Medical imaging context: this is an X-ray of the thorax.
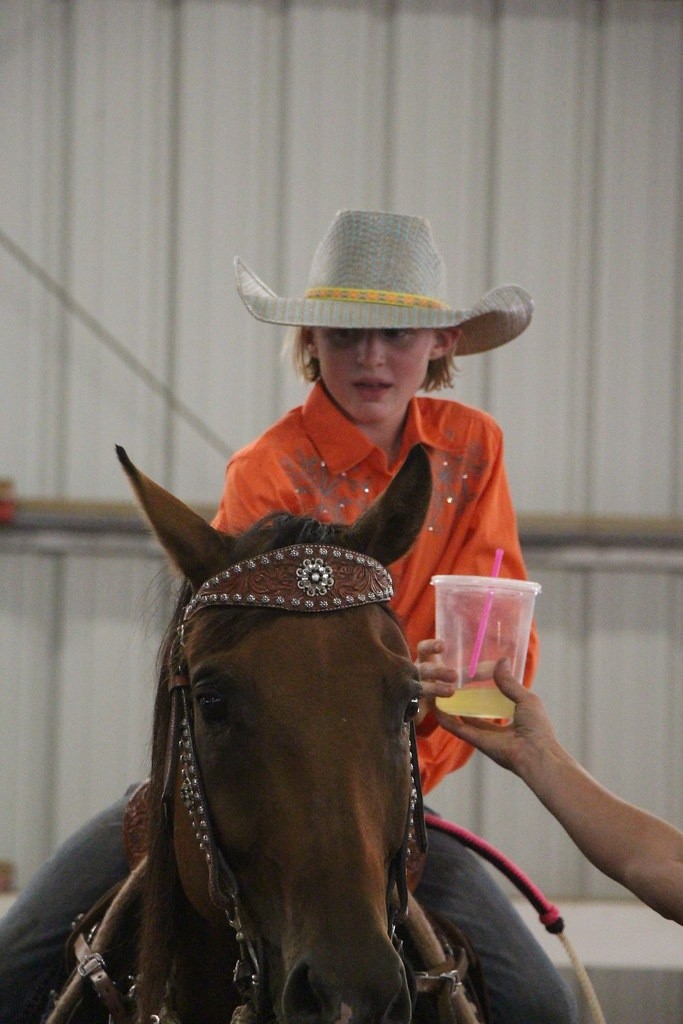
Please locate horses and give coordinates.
[37,445,485,1023]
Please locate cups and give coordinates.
[429,574,543,719]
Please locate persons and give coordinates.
[0,207,582,1024]
[426,658,683,929]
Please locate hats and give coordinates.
[234,212,534,357]
